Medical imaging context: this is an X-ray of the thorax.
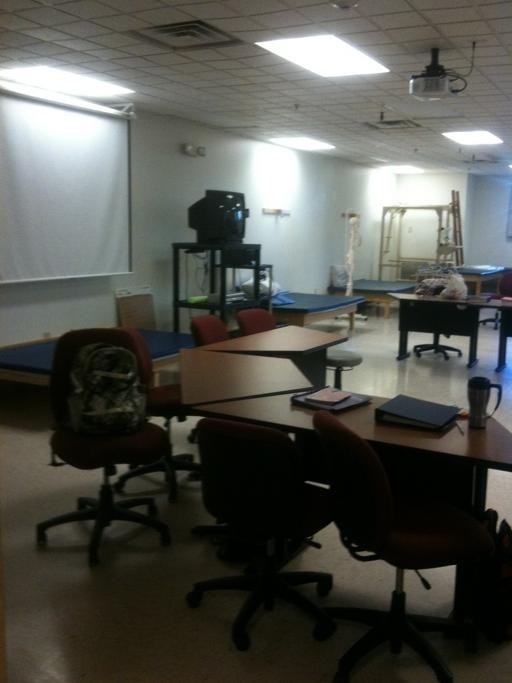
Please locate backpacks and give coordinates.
[68,343,145,435]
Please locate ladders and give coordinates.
[452,190,464,268]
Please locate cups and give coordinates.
[468,376,503,429]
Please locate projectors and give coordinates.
[407,76,454,102]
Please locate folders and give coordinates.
[375,395,463,431]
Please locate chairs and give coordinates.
[238,308,277,334]
[116,324,202,495]
[182,416,333,651]
[37,327,170,566]
[311,408,495,682]
[192,314,230,345]
[478,273,512,330]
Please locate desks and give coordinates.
[178,348,314,481]
[414,279,463,360]
[205,325,348,389]
[387,292,512,372]
[458,264,506,295]
[188,390,512,647]
[274,292,367,334]
[329,280,416,318]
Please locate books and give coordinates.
[307,384,352,405]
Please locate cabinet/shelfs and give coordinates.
[171,242,262,333]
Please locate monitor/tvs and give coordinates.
[187,189,250,243]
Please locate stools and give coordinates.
[324,350,364,390]
